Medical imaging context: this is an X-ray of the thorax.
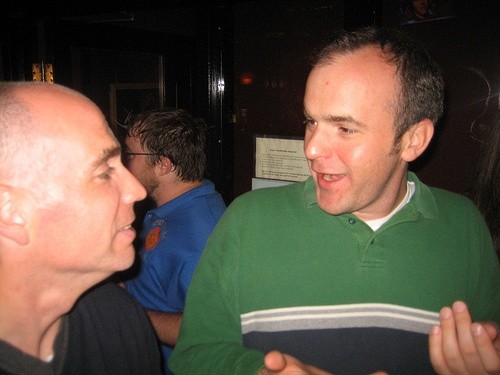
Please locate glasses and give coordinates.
[122,150,174,166]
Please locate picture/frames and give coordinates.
[107,81,164,140]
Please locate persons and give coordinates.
[0,80,167,375]
[165,27,500,375]
[118,108,227,360]
